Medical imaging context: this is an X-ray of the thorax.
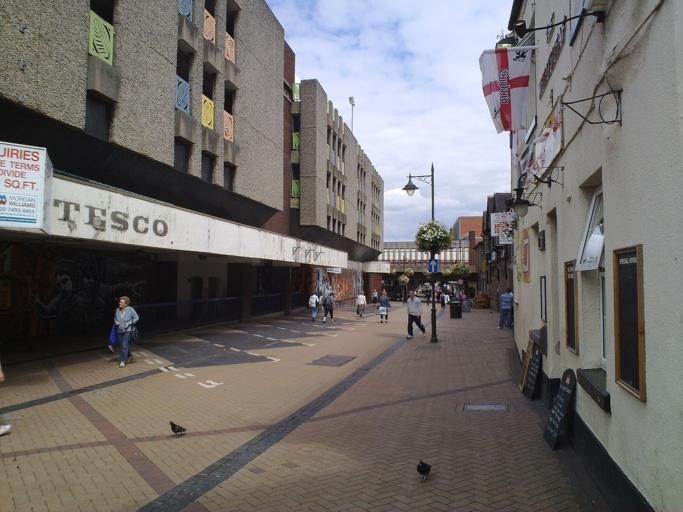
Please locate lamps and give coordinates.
[510,186,542,218]
[513,9,605,39]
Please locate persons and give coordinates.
[107,294,140,368]
[372,288,378,308]
[0,363,12,437]
[406,289,426,339]
[355,291,366,318]
[436,289,468,307]
[308,292,319,322]
[319,293,337,322]
[497,287,513,331]
[376,293,390,323]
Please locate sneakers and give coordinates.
[108,344,132,367]
[0,425,11,435]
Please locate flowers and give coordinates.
[415,219,451,255]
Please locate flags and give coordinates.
[477,46,535,135]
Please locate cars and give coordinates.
[419,283,439,296]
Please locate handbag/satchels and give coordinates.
[130,327,139,340]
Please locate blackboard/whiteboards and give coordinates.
[523,345,542,400]
[544,369,576,450]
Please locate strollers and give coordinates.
[460,300,469,312]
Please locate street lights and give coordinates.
[348,95,356,135]
[401,161,438,344]
[456,279,464,314]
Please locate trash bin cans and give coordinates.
[449,301,462,318]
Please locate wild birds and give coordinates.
[417,461,432,482]
[168,421,186,437]
